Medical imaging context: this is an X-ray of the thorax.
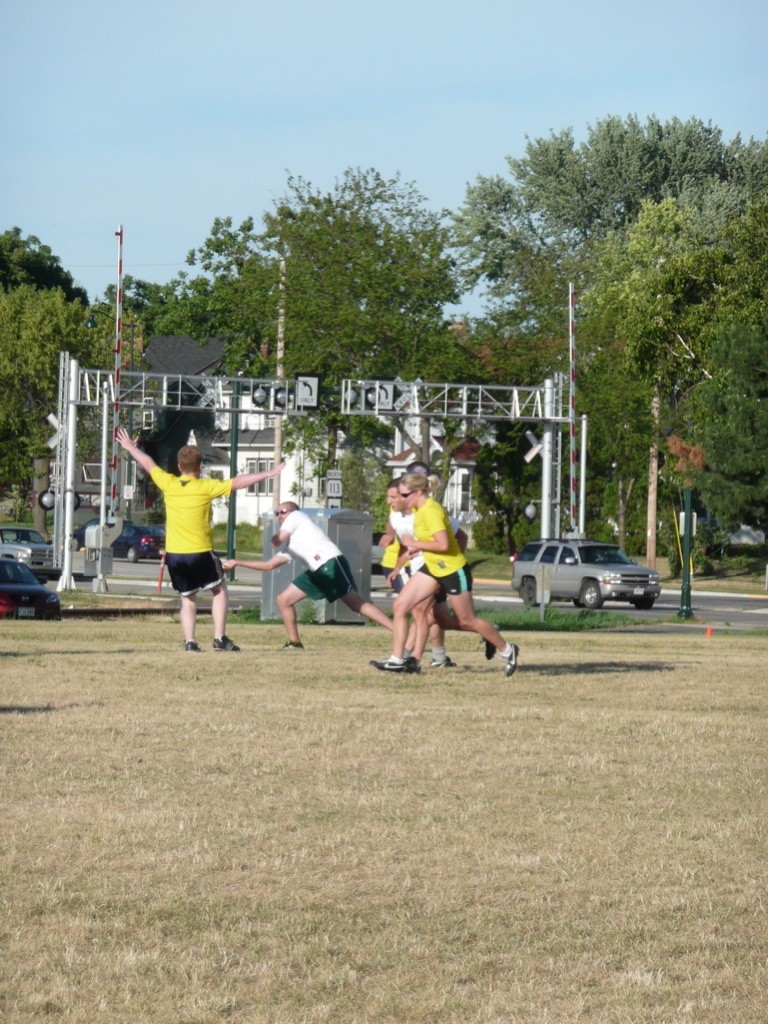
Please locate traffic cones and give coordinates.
[705,627,713,639]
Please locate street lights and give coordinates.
[86,308,139,520]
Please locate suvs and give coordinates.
[511,538,662,609]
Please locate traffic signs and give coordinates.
[296,375,318,407]
[374,382,392,410]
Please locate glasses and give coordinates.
[276,509,294,516]
[400,489,420,497]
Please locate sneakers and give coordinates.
[186,642,200,651]
[282,641,303,650]
[369,660,406,672]
[405,657,420,672]
[504,644,520,676]
[214,636,239,650]
[429,657,456,667]
[480,625,499,659]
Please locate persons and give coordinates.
[223,502,393,648]
[370,461,519,677]
[114,427,285,652]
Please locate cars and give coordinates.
[71,518,166,562]
[0,528,61,584]
[0,558,61,620]
[706,524,766,559]
